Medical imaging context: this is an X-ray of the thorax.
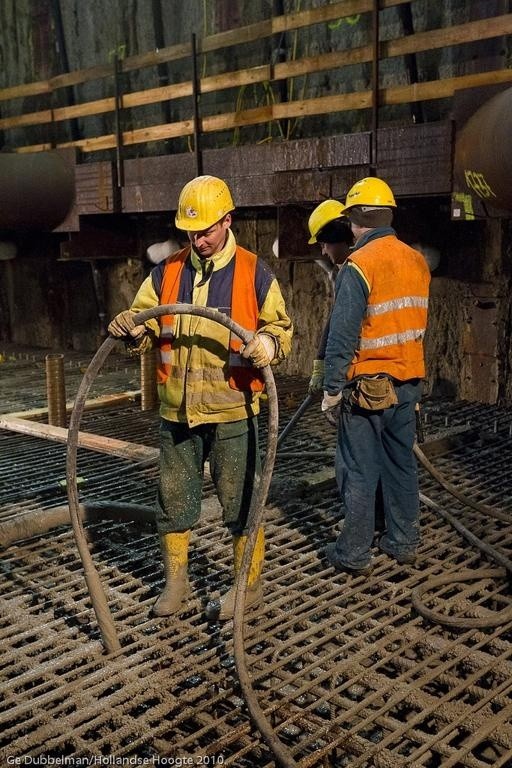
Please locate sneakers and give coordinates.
[372,530,417,564]
[325,542,373,577]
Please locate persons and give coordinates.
[104,173,297,621]
[317,174,430,574]
[302,196,395,505]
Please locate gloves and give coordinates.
[320,391,342,426]
[308,360,324,394]
[108,310,145,340]
[240,334,276,369]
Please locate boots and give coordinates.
[152,529,191,617]
[205,526,264,620]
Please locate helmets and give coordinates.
[308,199,345,245]
[340,176,397,214]
[174,175,235,232]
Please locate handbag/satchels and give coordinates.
[351,377,399,411]
